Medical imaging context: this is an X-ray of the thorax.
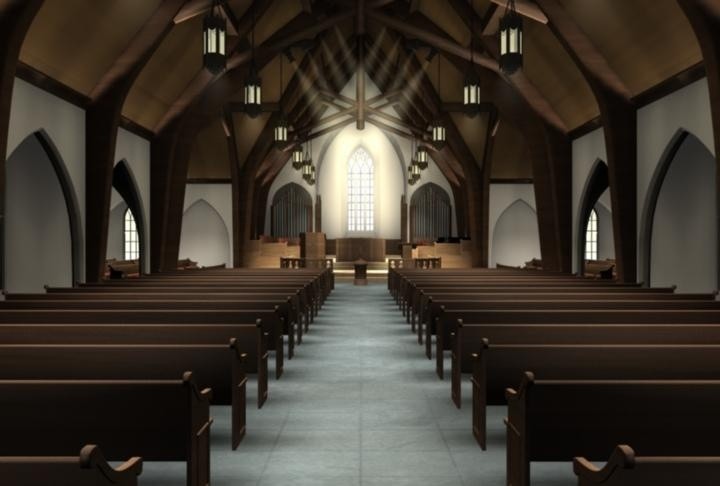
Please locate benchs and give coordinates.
[0,257,335,485]
[390,258,719,486]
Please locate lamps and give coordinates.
[293,87,316,185]
[204,2,228,78]
[272,48,289,147]
[244,1,262,118]
[464,11,482,118]
[499,0,523,76]
[432,53,448,149]
[407,90,429,186]
